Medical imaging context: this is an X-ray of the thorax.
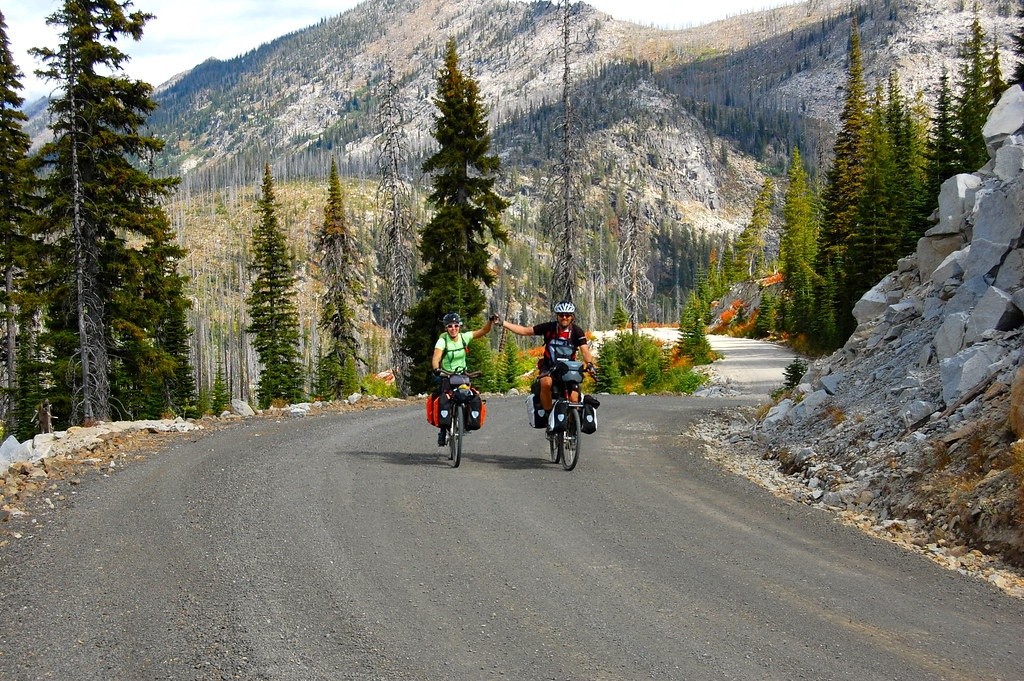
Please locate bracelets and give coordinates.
[587,362,593,365]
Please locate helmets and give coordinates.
[553,301,575,313]
[443,313,460,325]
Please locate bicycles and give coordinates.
[426,368,486,468]
[526,360,601,472]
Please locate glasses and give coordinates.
[557,312,573,318]
[445,324,459,328]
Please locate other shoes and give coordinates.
[437,430,447,446]
[545,425,556,440]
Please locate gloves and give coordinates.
[431,368,441,378]
[489,313,498,321]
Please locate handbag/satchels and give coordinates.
[525,393,548,428]
[581,393,600,434]
[426,390,452,429]
[462,387,486,431]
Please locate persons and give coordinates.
[494,301,596,450]
[431,312,499,446]
